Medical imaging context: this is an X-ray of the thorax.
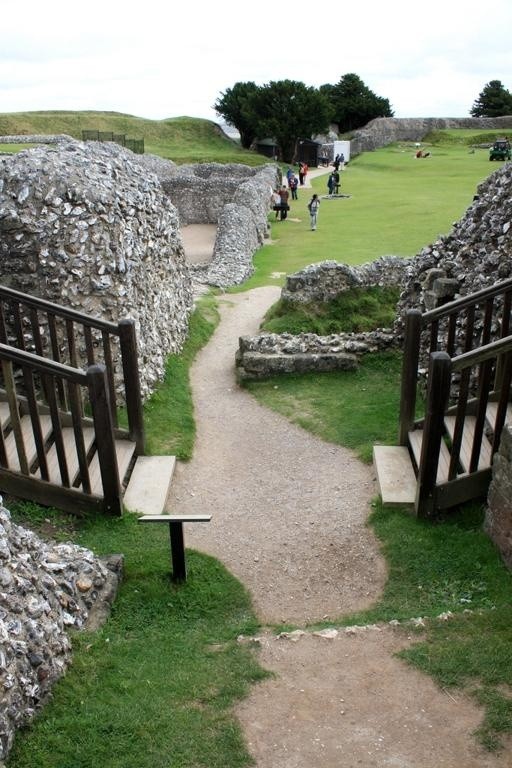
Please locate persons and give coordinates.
[271,161,308,221]
[327,153,345,194]
[307,194,321,231]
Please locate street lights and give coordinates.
[290,95,296,135]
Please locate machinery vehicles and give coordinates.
[489,139,512,160]
